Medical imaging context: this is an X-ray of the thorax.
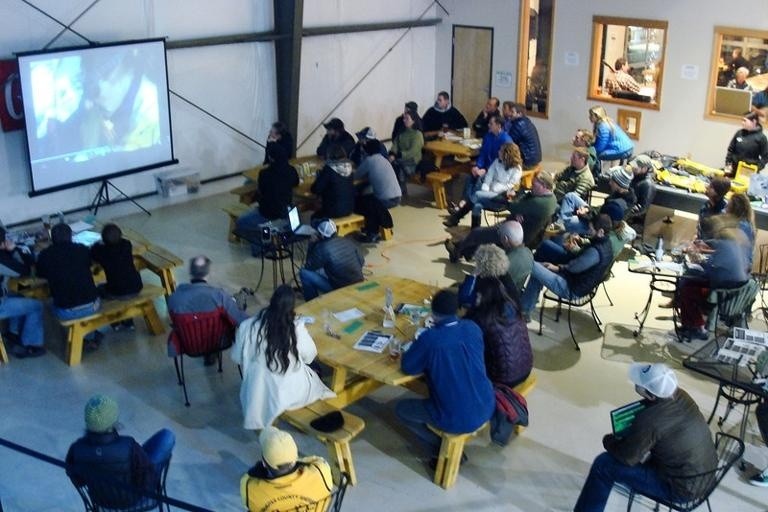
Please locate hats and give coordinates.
[84,393,120,434]
[311,218,338,239]
[256,425,298,474]
[629,154,653,170]
[612,164,634,190]
[628,361,679,398]
[321,118,345,131]
[600,200,625,222]
[355,126,376,141]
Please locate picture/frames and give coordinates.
[588,17,670,110]
[705,25,768,131]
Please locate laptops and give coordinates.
[288,206,319,236]
[611,399,659,463]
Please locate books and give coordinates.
[715,326,768,379]
[68,221,94,234]
[352,331,394,354]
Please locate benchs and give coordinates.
[231,182,257,205]
[426,374,538,489]
[122,226,183,297]
[0,334,10,363]
[282,400,365,486]
[415,168,452,209]
[330,213,391,241]
[521,164,540,189]
[59,284,166,366]
[224,201,253,243]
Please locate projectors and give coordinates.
[258,223,273,245]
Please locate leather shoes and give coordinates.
[2,329,22,347]
[442,216,458,227]
[83,330,101,352]
[447,206,458,216]
[15,344,46,359]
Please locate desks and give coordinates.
[425,130,483,158]
[627,243,703,342]
[293,275,468,385]
[10,218,145,294]
[683,334,768,471]
[643,159,768,230]
[244,156,367,198]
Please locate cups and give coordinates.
[429,279,438,301]
[389,339,401,361]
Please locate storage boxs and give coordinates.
[154,166,200,198]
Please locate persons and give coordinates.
[724,111,768,176]
[695,176,731,241]
[606,59,641,96]
[229,284,339,430]
[240,426,334,512]
[502,101,513,125]
[573,363,718,512]
[235,145,299,259]
[498,221,535,297]
[629,155,656,219]
[522,213,614,324]
[262,121,294,165]
[589,105,634,161]
[388,111,425,206]
[64,49,161,163]
[443,143,523,230]
[391,101,440,184]
[446,116,514,217]
[748,401,768,489]
[674,216,749,341]
[463,279,534,386]
[352,139,402,242]
[65,394,176,509]
[421,91,469,141]
[533,201,623,264]
[658,193,756,315]
[396,290,496,471]
[563,199,637,242]
[36,224,102,352]
[472,98,500,140]
[470,243,522,320]
[444,169,557,263]
[316,118,356,159]
[0,228,46,359]
[508,103,542,171]
[350,126,389,196]
[727,66,752,93]
[299,218,364,302]
[166,256,244,368]
[572,129,602,185]
[90,224,143,333]
[553,147,595,205]
[545,165,635,233]
[752,86,768,117]
[724,48,753,80]
[310,144,354,223]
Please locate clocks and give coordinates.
[518,0,556,119]
[616,109,641,140]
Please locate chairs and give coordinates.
[713,279,756,346]
[169,313,243,407]
[590,249,623,325]
[74,453,172,512]
[286,473,350,512]
[483,207,509,226]
[538,256,615,350]
[625,433,745,511]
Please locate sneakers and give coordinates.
[202,359,218,367]
[238,286,251,312]
[113,323,137,332]
[428,452,468,471]
[751,470,768,489]
[678,326,710,341]
[353,231,380,242]
[446,236,471,263]
